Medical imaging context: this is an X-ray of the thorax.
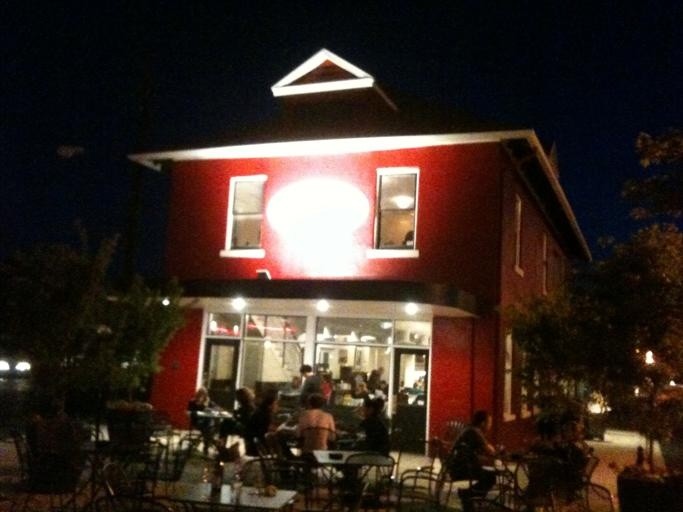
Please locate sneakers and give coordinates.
[458,488,471,509]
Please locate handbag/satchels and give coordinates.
[218,441,240,462]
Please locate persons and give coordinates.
[446,409,505,512]
[184,387,225,448]
[215,386,255,454]
[244,361,392,504]
[521,414,590,511]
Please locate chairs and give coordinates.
[6,403,601,511]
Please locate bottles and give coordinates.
[211,457,224,491]
